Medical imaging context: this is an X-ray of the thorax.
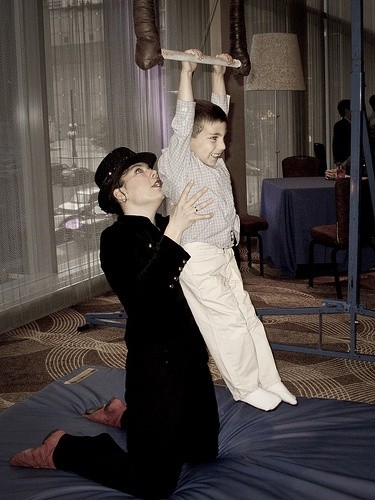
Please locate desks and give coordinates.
[258,176,375,278]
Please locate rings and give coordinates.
[195,206,198,211]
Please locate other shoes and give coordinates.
[84,397,126,428]
[10,428,66,469]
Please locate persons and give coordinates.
[369,95,375,137]
[157,49,297,410]
[332,99,351,175]
[8,146,219,500]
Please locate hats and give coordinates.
[93,146,157,218]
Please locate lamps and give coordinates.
[244,32,306,178]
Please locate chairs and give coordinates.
[230,178,268,276]
[280,156,324,179]
[308,178,375,300]
